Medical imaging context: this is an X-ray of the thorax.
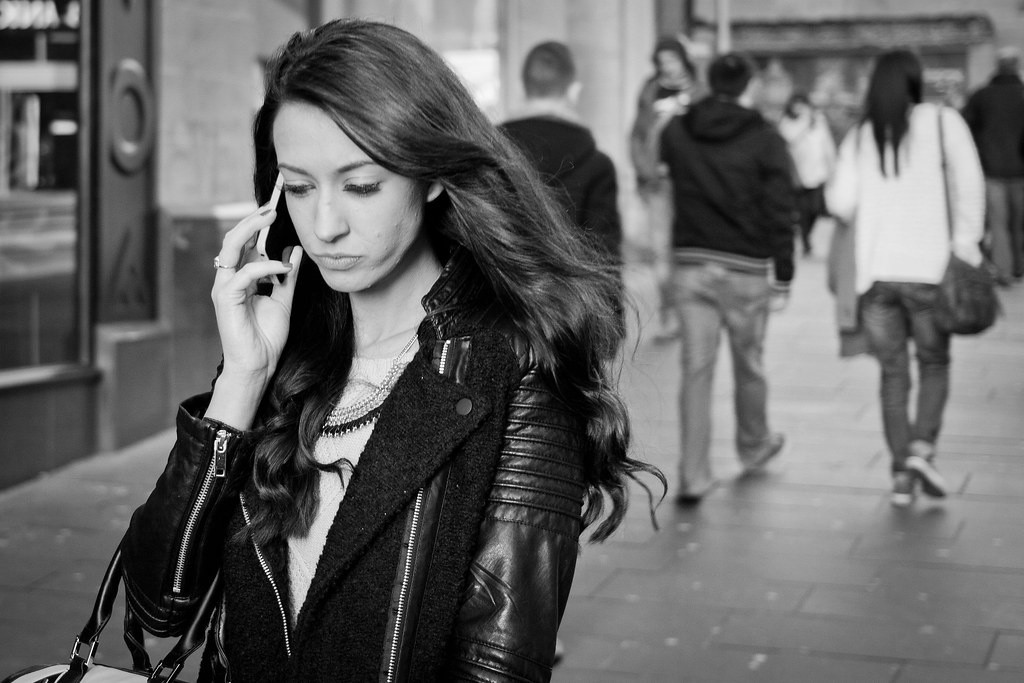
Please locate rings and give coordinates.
[214,257,239,270]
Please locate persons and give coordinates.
[823,50,987,505]
[124,19,636,683]
[658,54,794,505]
[488,42,626,362]
[960,46,1024,282]
[631,34,713,345]
[779,94,836,257]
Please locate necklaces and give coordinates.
[319,335,419,437]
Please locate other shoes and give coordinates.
[741,431,785,474]
[890,476,918,506]
[677,478,718,506]
[905,444,950,496]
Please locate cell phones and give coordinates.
[257,171,300,287]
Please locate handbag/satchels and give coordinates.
[3,512,218,683]
[936,251,1003,331]
